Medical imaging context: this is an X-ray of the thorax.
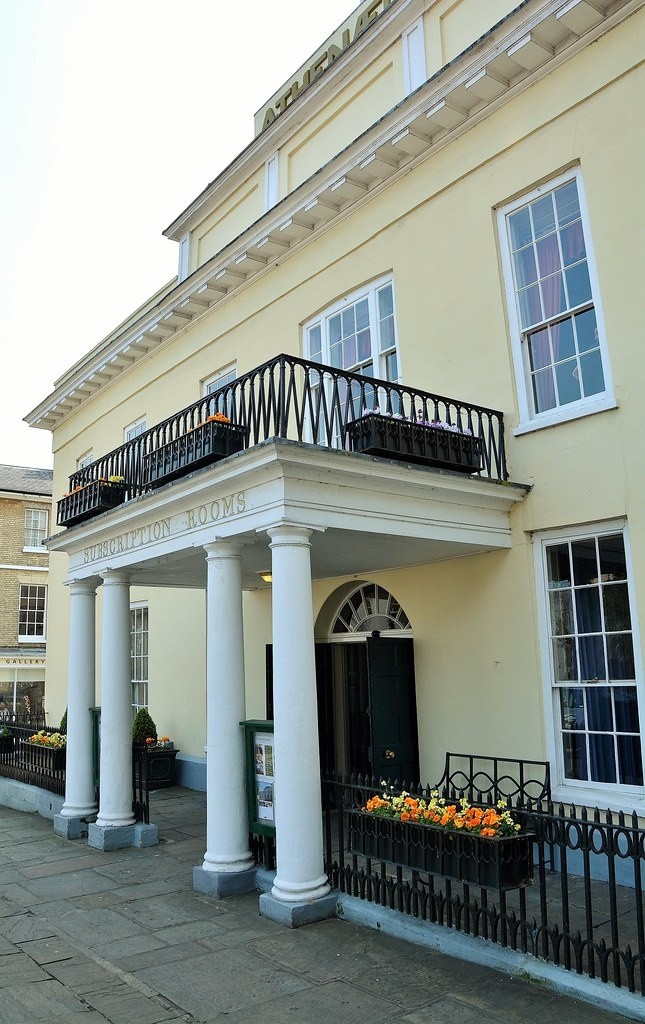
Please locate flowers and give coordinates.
[143,736,173,751]
[63,475,125,498]
[360,404,472,436]
[168,412,231,451]
[26,730,66,749]
[362,781,522,837]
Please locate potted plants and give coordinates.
[0,723,14,754]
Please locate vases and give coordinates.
[346,413,485,473]
[141,419,249,489]
[56,479,131,527]
[347,808,535,892]
[19,741,66,771]
[134,750,180,790]
